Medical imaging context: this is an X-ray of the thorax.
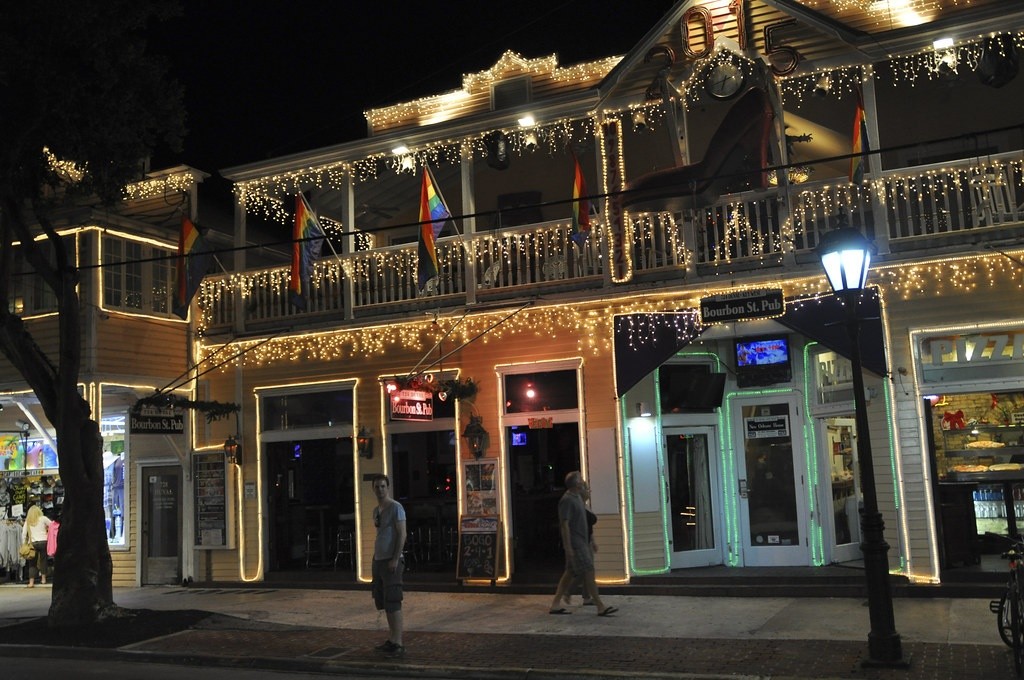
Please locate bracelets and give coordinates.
[799,135,802,143]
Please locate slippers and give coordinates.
[584,600,596,605]
[376,641,404,652]
[598,607,618,616]
[549,609,572,615]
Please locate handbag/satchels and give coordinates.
[20,543,36,560]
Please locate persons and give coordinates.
[564,480,599,605]
[42,440,58,467]
[26,442,42,468]
[47,510,62,555]
[548,472,619,615]
[22,504,52,587]
[112,452,124,542]
[768,124,814,163]
[14,445,25,470]
[103,451,119,543]
[371,473,406,658]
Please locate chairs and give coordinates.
[403,527,458,563]
[304,533,354,571]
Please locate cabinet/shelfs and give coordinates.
[943,427,1024,482]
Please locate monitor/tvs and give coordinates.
[733,335,793,388]
[512,433,526,446]
[667,372,726,409]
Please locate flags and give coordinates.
[758,75,775,191]
[288,187,325,311]
[417,166,449,291]
[848,87,869,194]
[176,215,199,308]
[569,160,591,248]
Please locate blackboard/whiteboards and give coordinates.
[456,514,500,580]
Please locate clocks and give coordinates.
[705,60,746,100]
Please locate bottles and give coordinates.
[970,486,1024,518]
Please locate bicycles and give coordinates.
[985,531,1024,680]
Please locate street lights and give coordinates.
[815,201,914,669]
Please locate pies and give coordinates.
[967,441,1005,447]
[953,463,1021,471]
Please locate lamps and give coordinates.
[357,426,373,460]
[439,387,489,461]
[16,419,29,431]
[224,435,242,465]
